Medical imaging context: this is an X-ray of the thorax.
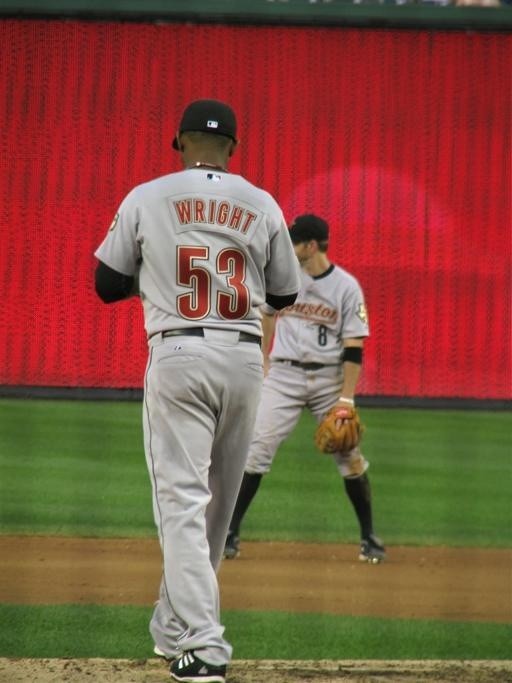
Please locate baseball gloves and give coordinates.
[315,404,362,454]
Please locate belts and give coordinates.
[162,328,261,344]
[277,360,325,370]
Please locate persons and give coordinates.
[93,99,302,682]
[223,212,387,565]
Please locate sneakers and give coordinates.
[221,531,241,559]
[153,645,184,662]
[359,534,386,564]
[169,649,226,683]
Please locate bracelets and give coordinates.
[339,396,354,406]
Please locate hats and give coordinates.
[173,100,237,152]
[289,215,328,240]
